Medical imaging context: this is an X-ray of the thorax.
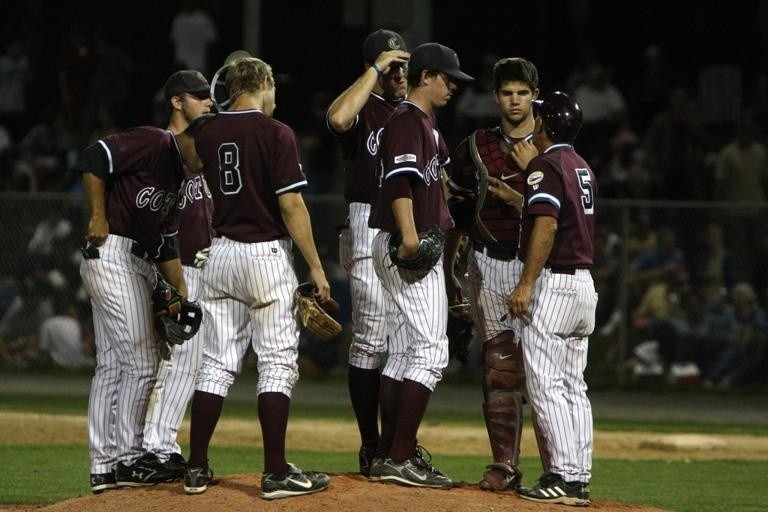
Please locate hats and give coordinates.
[410,42,476,83]
[613,132,636,145]
[163,70,211,103]
[362,29,408,66]
[532,90,585,143]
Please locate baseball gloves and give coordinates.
[154,286,201,345]
[389,229,444,270]
[294,284,343,339]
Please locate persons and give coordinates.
[436,236,488,388]
[325,27,456,477]
[375,44,466,490]
[183,57,333,497]
[445,55,570,492]
[507,97,597,505]
[293,254,352,378]
[585,66,768,388]
[0,32,121,369]
[141,72,222,477]
[77,120,193,493]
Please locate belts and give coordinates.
[471,241,517,261]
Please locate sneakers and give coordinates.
[89,470,116,493]
[183,464,214,494]
[116,453,179,487]
[162,453,187,481]
[357,443,457,490]
[476,462,590,507]
[259,463,330,500]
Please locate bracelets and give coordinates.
[369,63,386,82]
